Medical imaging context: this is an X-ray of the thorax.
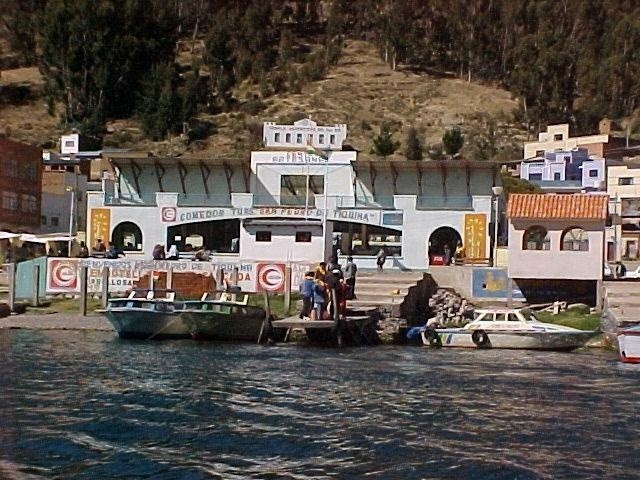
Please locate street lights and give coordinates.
[492,186,503,267]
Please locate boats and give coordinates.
[93,288,266,341]
[419,307,604,349]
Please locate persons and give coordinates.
[455,240,466,258]
[153,242,209,261]
[444,245,452,266]
[77,239,118,258]
[377,248,386,271]
[299,255,358,320]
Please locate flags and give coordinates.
[307,140,329,161]
